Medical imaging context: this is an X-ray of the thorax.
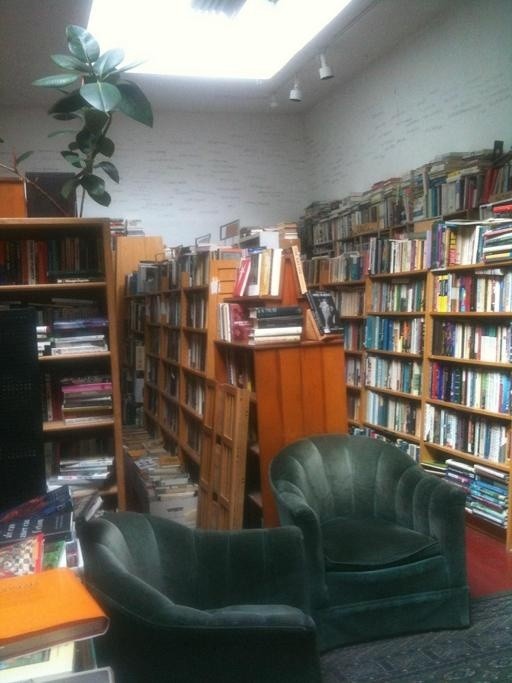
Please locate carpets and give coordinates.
[324,591,512,683]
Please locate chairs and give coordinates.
[268,434,471,648]
[77,510,321,683]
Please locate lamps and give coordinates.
[319,52,334,80]
[289,77,302,102]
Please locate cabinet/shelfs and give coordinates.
[0,162,512,557]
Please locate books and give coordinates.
[1,149,512,682]
